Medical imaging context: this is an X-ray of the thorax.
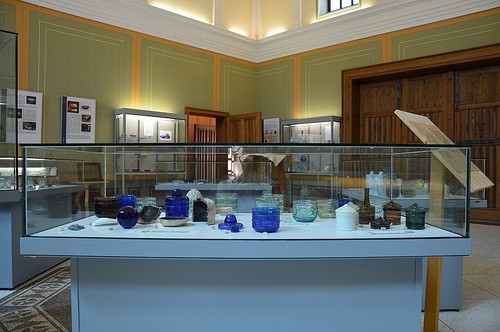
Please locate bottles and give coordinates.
[192,197,208,221]
[376,171,384,196]
[359,187,375,224]
[165,188,190,217]
[366,171,378,195]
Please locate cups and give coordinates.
[292,199,318,222]
[252,193,284,233]
[120,194,136,207]
[214,192,239,216]
[93,196,120,218]
